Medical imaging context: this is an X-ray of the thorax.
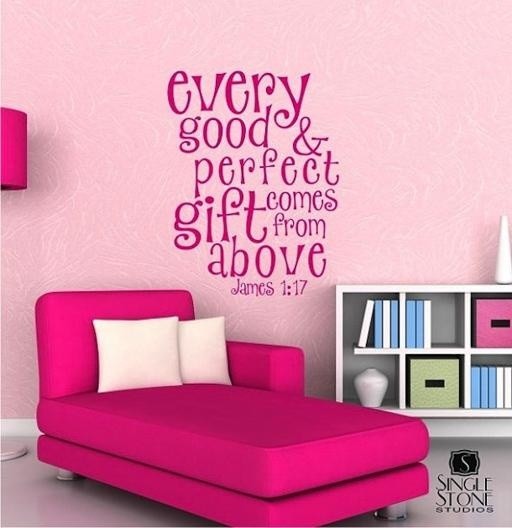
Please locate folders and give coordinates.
[407,298,430,348]
[357,299,397,348]
[471,366,511,408]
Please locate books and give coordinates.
[357,299,432,352]
[471,364,512,408]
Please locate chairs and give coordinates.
[34,288,431,527]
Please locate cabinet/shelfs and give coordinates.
[335,286,512,522]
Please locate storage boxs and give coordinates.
[473,299,512,348]
[409,358,460,409]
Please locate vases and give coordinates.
[354,368,389,408]
[495,216,511,284]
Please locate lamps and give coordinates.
[0,107,28,462]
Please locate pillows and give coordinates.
[92,315,184,394]
[178,316,233,386]
